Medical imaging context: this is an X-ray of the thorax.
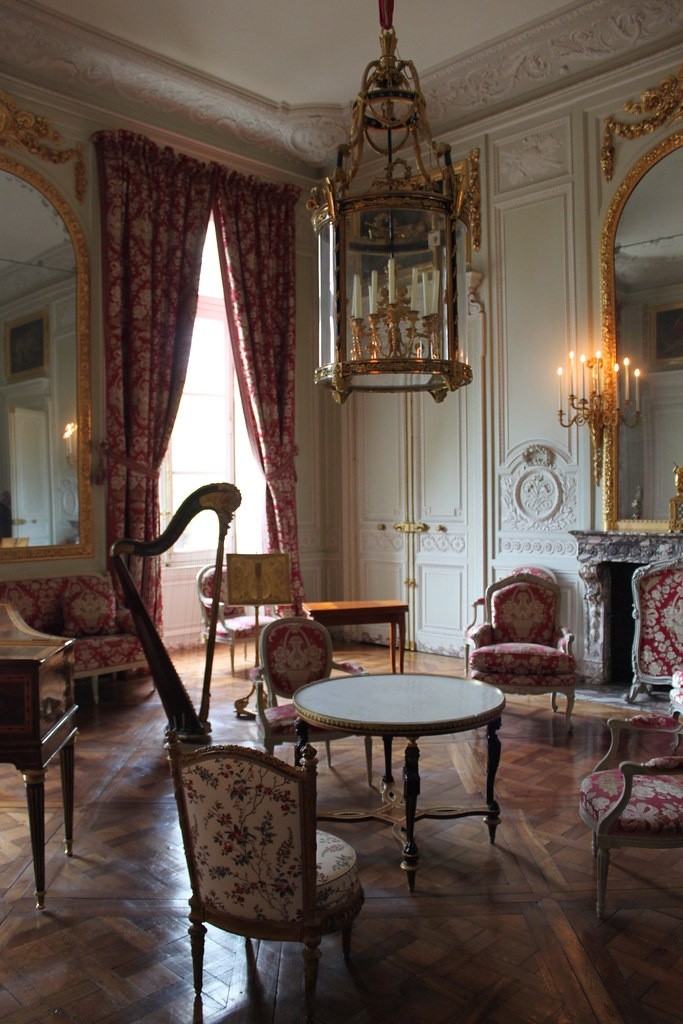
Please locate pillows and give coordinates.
[61,589,120,637]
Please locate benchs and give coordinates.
[0,572,157,705]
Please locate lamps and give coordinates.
[556,349,641,487]
[63,421,80,467]
[305,0,473,403]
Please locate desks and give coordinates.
[302,600,409,674]
[292,673,506,895]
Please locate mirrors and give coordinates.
[600,128,683,535]
[1,157,94,562]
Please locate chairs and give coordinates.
[254,616,373,787]
[196,564,283,678]
[464,564,577,733]
[164,730,366,1024]
[580,711,683,918]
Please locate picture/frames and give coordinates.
[356,159,469,287]
[4,305,49,380]
[652,302,683,365]
